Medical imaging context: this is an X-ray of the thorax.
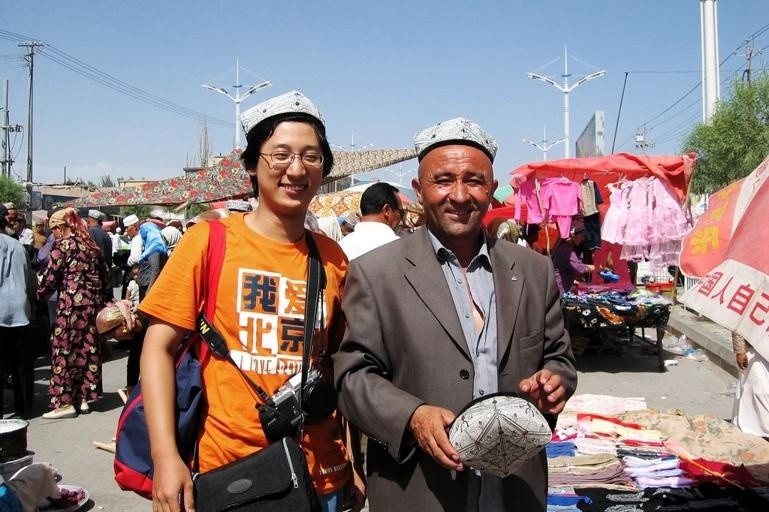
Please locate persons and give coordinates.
[186,200,253,233]
[0,200,185,422]
[330,119,578,511]
[136,89,368,512]
[549,226,604,297]
[303,177,532,265]
[730,329,769,442]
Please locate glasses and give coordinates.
[259,152,325,166]
[392,207,404,217]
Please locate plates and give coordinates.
[41,483,88,511]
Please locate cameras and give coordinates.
[258,368,338,445]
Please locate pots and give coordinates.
[0,417,31,458]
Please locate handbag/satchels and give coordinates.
[182,438,324,512]
[114,346,204,501]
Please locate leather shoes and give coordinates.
[77,403,90,415]
[43,405,77,419]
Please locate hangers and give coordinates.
[604,172,627,189]
[582,170,588,182]
[560,170,569,182]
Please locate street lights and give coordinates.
[522,125,568,160]
[529,44,608,160]
[200,61,272,157]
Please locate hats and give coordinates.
[3,200,251,232]
[448,393,553,478]
[240,90,327,143]
[413,118,497,164]
